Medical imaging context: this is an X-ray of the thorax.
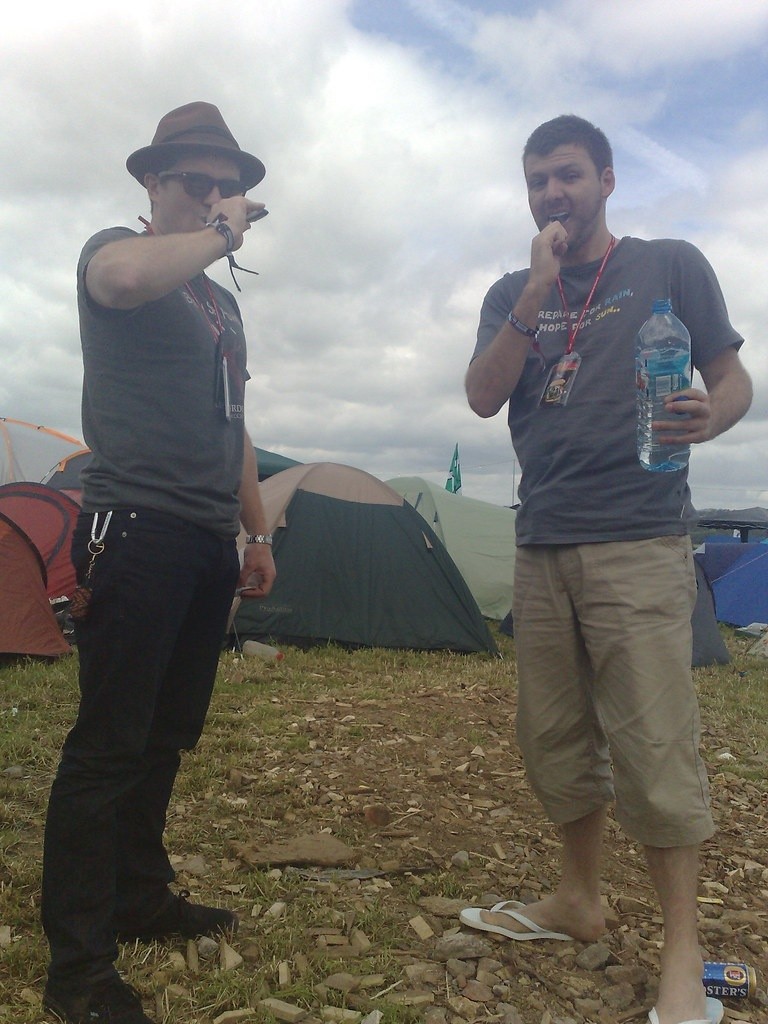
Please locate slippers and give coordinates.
[645,997,725,1024]
[458,900,576,941]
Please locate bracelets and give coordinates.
[208,213,260,294]
[246,534,273,544]
[507,311,539,339]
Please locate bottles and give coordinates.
[635,298,691,473]
[243,640,283,661]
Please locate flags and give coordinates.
[445,443,462,494]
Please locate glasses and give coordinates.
[157,170,251,199]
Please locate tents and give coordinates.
[0,416,768,671]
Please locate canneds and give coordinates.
[701,961,756,998]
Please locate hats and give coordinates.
[125,100,266,192]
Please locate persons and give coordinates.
[44,103,278,1022]
[465,116,752,1023]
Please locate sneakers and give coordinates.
[40,973,158,1024]
[110,888,240,946]
